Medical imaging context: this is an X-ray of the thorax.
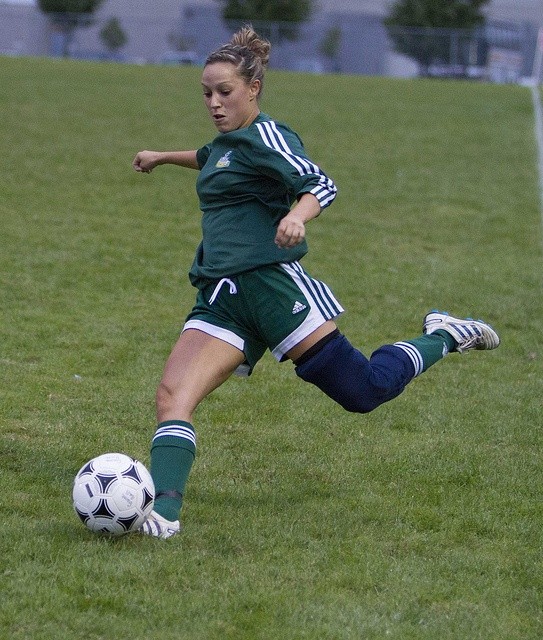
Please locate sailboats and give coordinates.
[72,453,155,536]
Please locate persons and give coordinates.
[130,22,501,540]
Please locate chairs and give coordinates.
[422,308,500,354]
[136,511,181,540]
